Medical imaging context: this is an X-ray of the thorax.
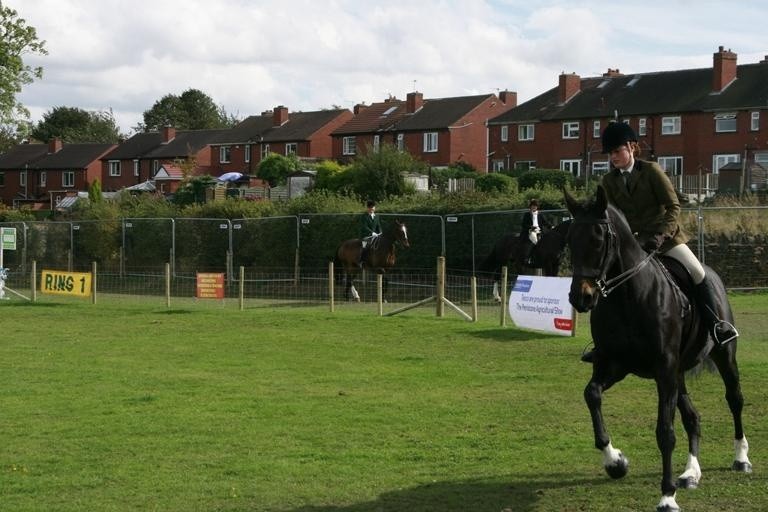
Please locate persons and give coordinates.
[359,201,383,264]
[519,200,554,264]
[579,123,739,362]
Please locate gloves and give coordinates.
[644,234,661,251]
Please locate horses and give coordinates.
[559,183,754,512]
[480,217,574,304]
[333,218,412,304]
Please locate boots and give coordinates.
[696,275,736,338]
[358,248,368,267]
[579,348,599,362]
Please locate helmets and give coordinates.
[529,200,539,207]
[367,200,377,207]
[601,123,638,153]
